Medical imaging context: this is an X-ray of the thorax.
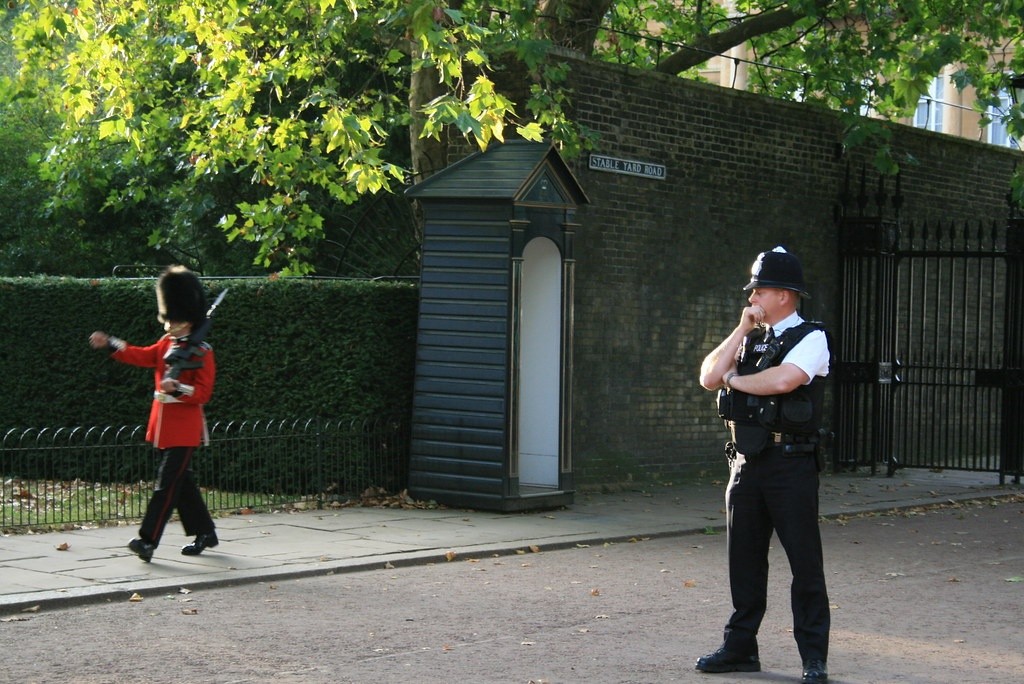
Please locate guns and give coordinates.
[807,435,827,472]
[160,286,231,398]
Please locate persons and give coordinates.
[89,321,218,562]
[695,252,837,684]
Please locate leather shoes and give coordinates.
[800,658,827,684]
[696,645,762,672]
[130,539,153,562]
[181,530,218,556]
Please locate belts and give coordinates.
[772,433,819,444]
[154,390,181,404]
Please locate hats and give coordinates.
[157,266,206,331]
[742,248,811,300]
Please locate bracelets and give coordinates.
[727,373,739,385]
